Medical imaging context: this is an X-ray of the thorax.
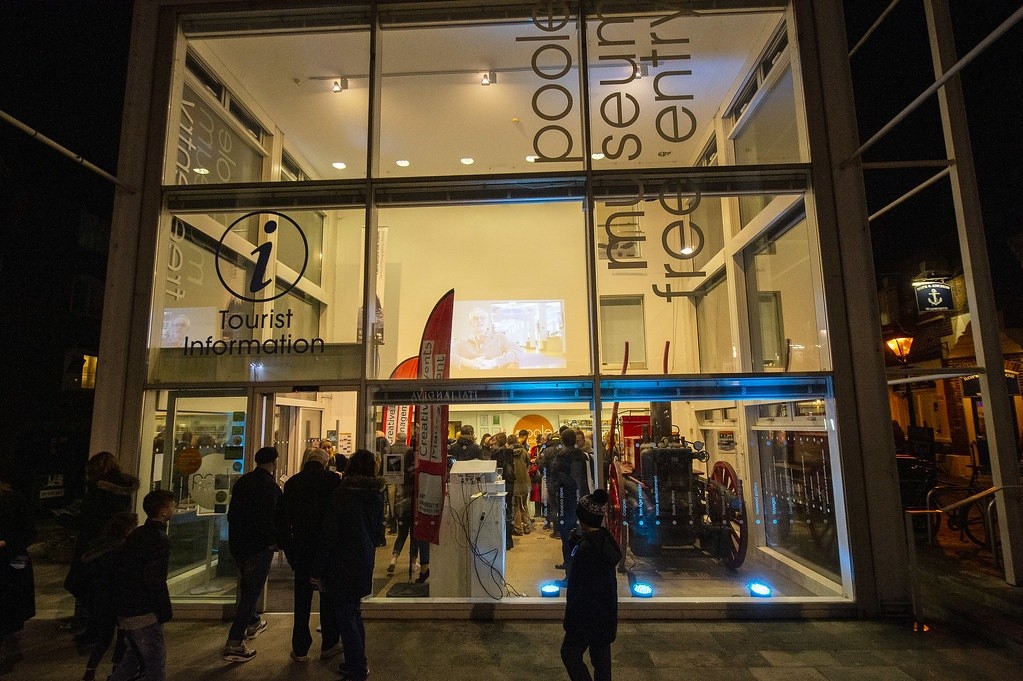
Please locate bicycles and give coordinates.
[911,460,1003,549]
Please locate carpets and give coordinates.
[268,578,391,611]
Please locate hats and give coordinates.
[575,489,609,528]
[306,448,329,461]
[255,446,279,464]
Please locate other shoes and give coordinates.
[337,672,367,681]
[554,577,568,588]
[415,567,430,583]
[337,662,369,675]
[320,643,344,659]
[290,649,308,662]
[72,628,106,651]
[543,523,562,539]
[554,562,566,569]
[511,518,537,536]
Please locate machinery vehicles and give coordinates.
[609,339,750,569]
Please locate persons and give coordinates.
[0,450,174,681]
[559,489,621,681]
[150,423,231,513]
[453,307,523,369]
[220,439,390,681]
[892,419,905,452]
[377,424,622,585]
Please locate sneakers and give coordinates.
[409,562,421,571]
[386,561,396,573]
[223,640,257,663]
[246,620,268,639]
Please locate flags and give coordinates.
[382,353,421,447]
[414,287,454,545]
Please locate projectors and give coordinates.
[450,460,498,484]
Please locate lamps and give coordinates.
[634,64,649,79]
[540,585,560,597]
[333,77,349,93]
[482,71,497,86]
[632,583,653,598]
[749,583,772,597]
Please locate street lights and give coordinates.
[887,323,917,425]
